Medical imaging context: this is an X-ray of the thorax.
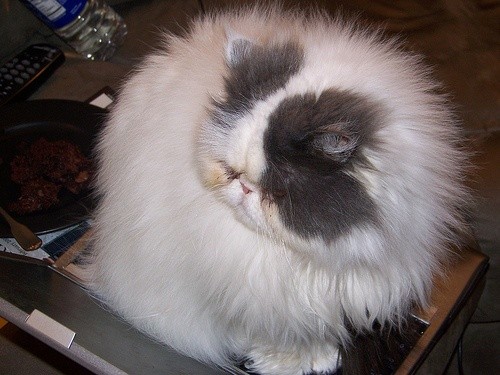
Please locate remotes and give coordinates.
[1,43,65,111]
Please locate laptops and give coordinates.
[0,222,488,375]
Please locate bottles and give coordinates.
[20,0,129,64]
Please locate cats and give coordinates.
[60,0,483,375]
